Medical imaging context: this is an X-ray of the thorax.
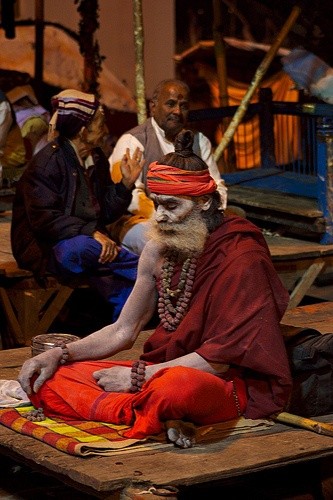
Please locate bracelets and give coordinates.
[25,407,46,422]
[130,360,148,392]
[52,341,70,365]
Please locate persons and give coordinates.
[17,130,293,448]
[11,89,147,324]
[109,78,228,257]
[0,88,26,190]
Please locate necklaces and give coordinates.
[158,251,197,332]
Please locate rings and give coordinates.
[136,158,141,163]
